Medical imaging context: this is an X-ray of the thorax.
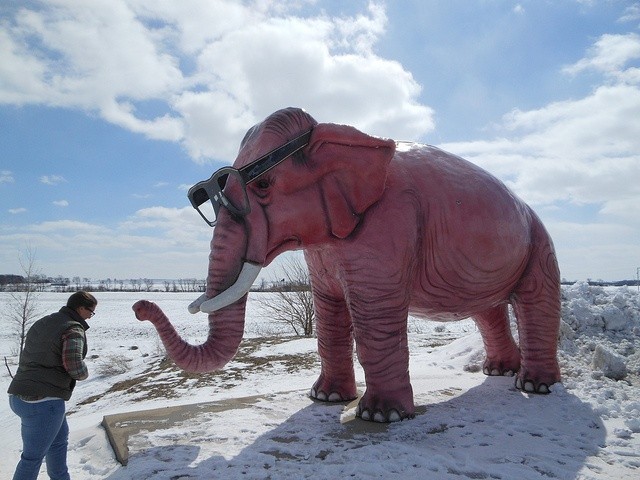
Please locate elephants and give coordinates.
[132,107,561,423]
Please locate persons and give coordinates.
[7,289,98,480]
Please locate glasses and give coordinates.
[86,308,96,317]
